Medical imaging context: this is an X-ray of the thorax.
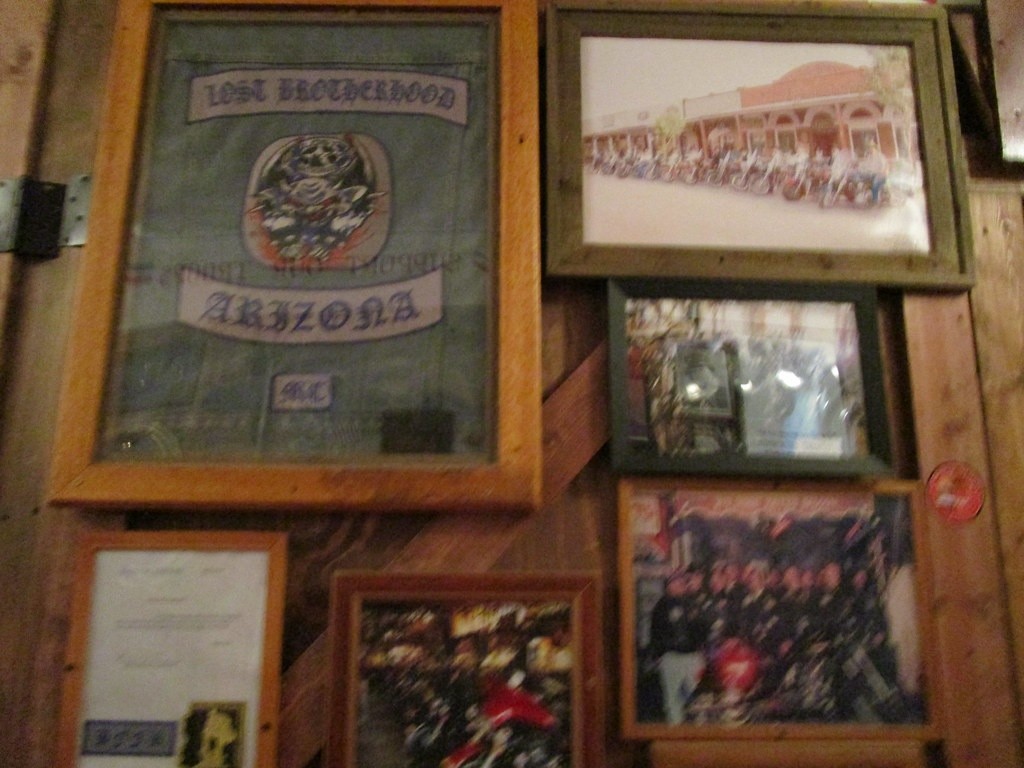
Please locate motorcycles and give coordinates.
[371,656,571,767]
[586,145,893,211]
[707,634,842,724]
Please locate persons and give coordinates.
[649,509,927,726]
[365,604,573,768]
[589,139,890,207]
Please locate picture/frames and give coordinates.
[43,0,547,512]
[327,567,604,768]
[58,532,288,767]
[619,481,946,742]
[540,8,978,297]
[600,277,892,479]
